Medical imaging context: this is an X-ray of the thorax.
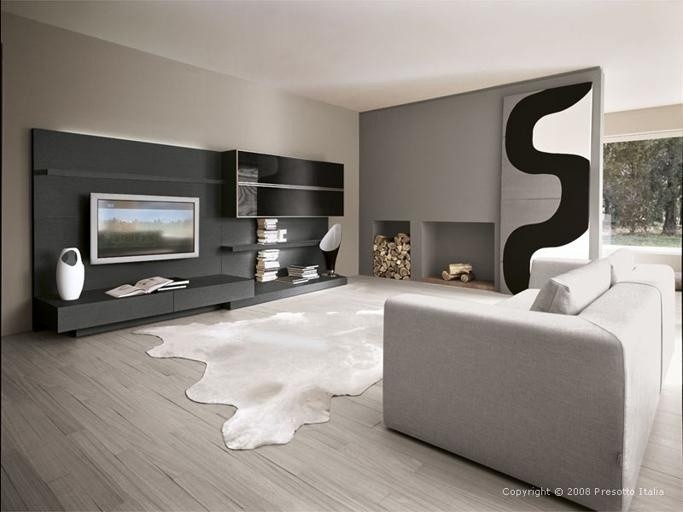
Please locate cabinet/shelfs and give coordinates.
[34,273,254,339]
[222,148,345,220]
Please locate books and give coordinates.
[105,275,190,299]
[287,264,319,285]
[254,219,286,281]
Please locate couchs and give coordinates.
[383,259,675,512]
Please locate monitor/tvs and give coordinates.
[90,193,200,265]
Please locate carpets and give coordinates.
[131,310,384,452]
